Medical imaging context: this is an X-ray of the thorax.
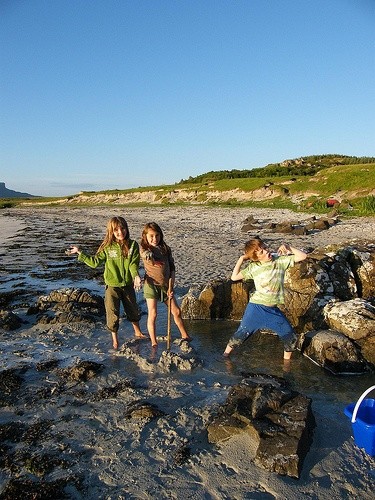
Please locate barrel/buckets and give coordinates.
[343,385,375,457]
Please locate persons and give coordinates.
[225,239,307,359]
[140,222,191,346]
[65,217,146,347]
